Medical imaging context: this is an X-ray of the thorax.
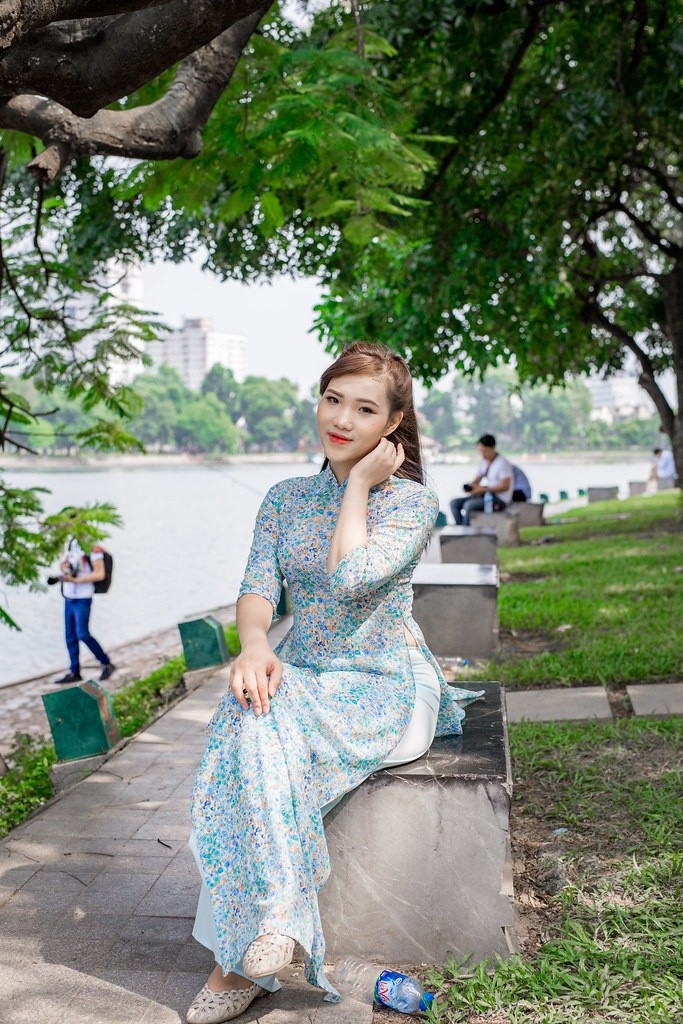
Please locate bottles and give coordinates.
[484,491,493,513]
[333,956,435,1013]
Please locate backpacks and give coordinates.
[512,465,531,502]
[86,544,113,593]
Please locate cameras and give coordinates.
[47,571,68,586]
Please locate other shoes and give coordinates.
[243,933,296,978]
[186,963,270,1024]
[100,663,116,680]
[55,672,82,683]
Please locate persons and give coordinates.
[184,341,487,1024]
[510,464,531,502]
[449,434,515,527]
[53,507,116,684]
[648,448,678,480]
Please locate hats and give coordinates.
[477,435,496,447]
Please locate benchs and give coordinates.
[629,482,646,496]
[290,681,514,968]
[440,524,498,564]
[469,511,520,547]
[658,479,673,490]
[508,500,545,527]
[588,485,619,503]
[411,561,500,660]
